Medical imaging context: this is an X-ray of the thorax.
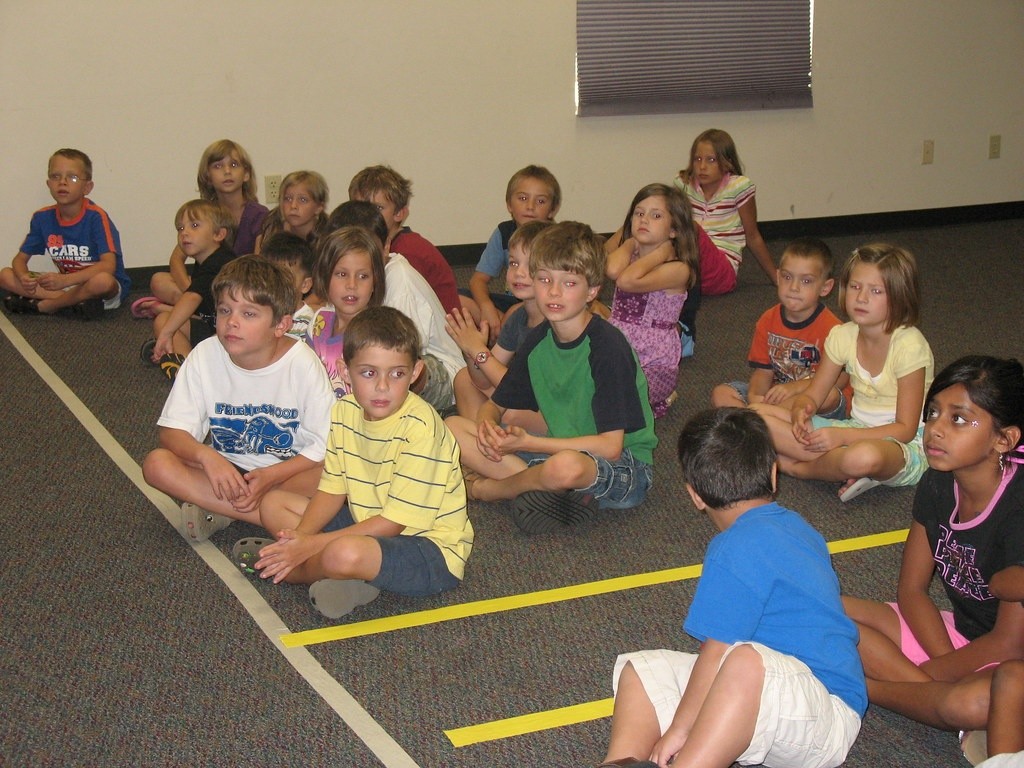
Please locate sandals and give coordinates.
[64,299,104,318]
[4,295,42,314]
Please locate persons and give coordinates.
[142,255,336,545]
[713,235,937,503]
[232,306,474,619]
[840,354,1023,768]
[130,139,700,535]
[599,128,778,297]
[1,149,131,317]
[592,407,871,768]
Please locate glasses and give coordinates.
[49,173,90,183]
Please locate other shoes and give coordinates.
[513,489,599,532]
[232,537,277,576]
[142,339,184,378]
[182,501,232,541]
[309,579,380,618]
[459,464,481,501]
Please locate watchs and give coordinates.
[474,351,494,370]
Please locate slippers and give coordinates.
[839,478,880,503]
[131,296,162,319]
